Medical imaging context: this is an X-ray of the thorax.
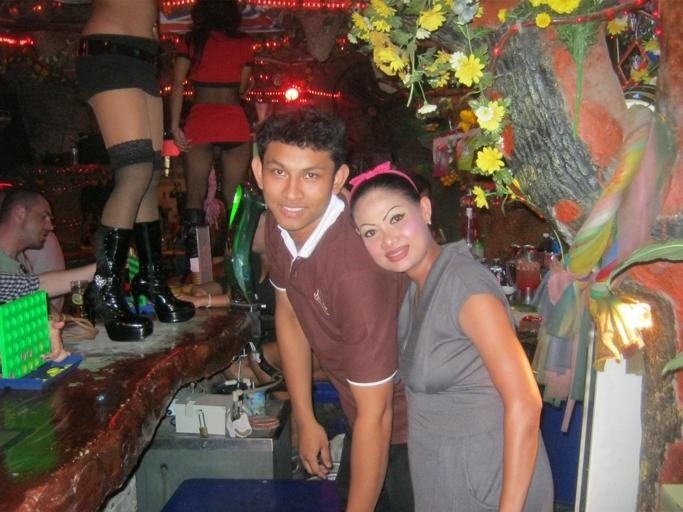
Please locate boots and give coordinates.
[83,223,153,341]
[181,209,206,284]
[131,219,195,323]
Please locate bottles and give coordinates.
[472,232,549,286]
[462,207,476,248]
[424,113,433,132]
[414,98,423,119]
[433,111,442,130]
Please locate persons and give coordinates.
[246,111,414,512]
[1,162,332,403]
[350,160,556,512]
[76,0,194,338]
[169,0,253,281]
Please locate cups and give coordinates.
[69,278,88,318]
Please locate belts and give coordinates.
[79,39,159,64]
